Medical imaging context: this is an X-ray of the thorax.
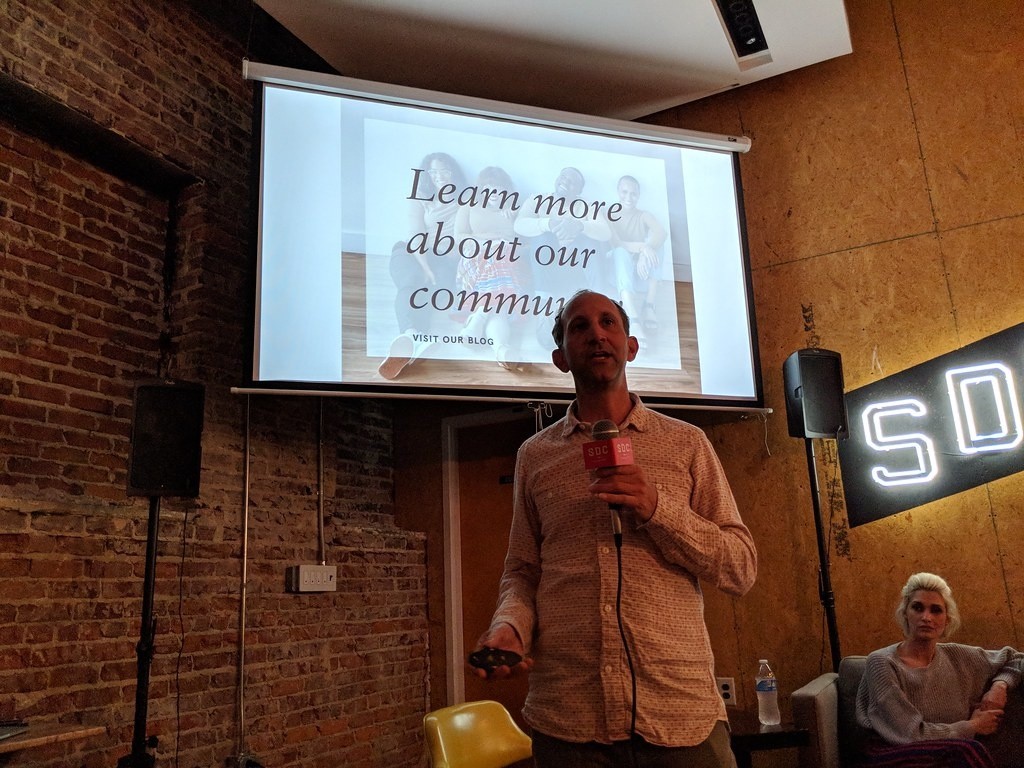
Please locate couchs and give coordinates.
[790,654,1024,768]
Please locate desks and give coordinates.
[725,709,813,768]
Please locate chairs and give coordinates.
[422,698,533,768]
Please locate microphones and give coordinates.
[581,419,634,546]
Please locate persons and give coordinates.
[379,151,667,380]
[856,572,1023,768]
[469,291,758,768]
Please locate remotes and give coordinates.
[469,646,522,667]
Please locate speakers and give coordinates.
[124,382,207,499]
[782,348,851,441]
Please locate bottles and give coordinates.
[756,659,781,725]
